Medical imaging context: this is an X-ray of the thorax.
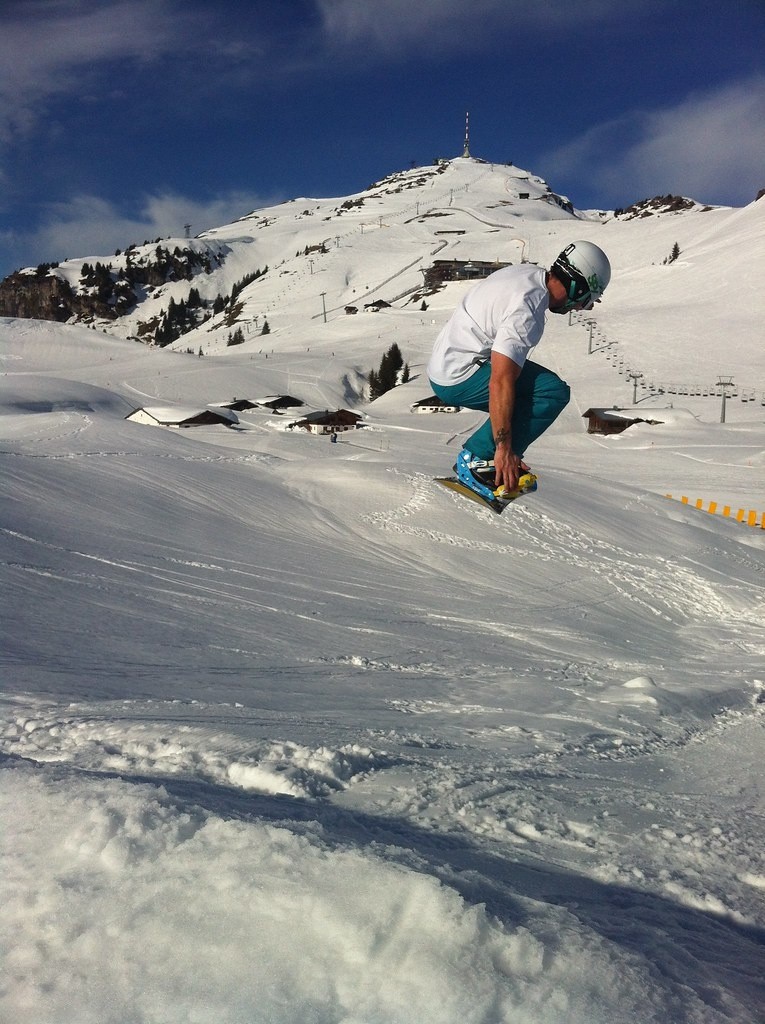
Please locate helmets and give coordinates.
[556,240,611,310]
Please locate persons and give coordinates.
[426,241,611,498]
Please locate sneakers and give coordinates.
[452,454,536,498]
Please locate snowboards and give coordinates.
[432,465,539,517]
